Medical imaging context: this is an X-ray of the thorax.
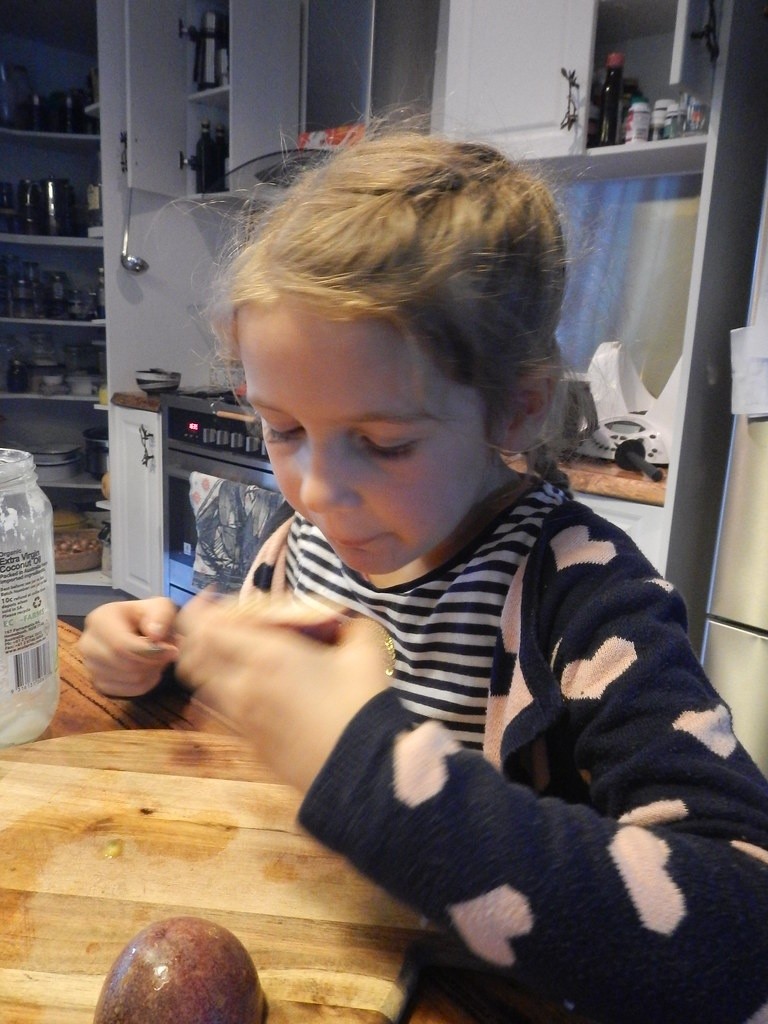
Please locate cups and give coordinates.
[0,176,74,217]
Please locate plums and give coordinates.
[91,917,263,1024]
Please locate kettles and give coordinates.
[545,380,598,458]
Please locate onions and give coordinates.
[54,535,101,553]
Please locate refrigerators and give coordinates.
[698,168,767,786]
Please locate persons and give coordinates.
[75,136,768,1023]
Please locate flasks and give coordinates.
[195,11,227,90]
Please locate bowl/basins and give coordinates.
[82,427,109,480]
[135,370,181,396]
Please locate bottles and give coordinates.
[663,103,681,140]
[217,124,229,194]
[0,447,65,745]
[649,98,677,141]
[597,48,628,149]
[196,120,217,194]
[625,102,652,144]
[0,254,105,322]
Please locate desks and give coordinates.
[46,621,603,1024]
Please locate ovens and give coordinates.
[161,390,306,600]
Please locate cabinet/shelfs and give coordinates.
[109,404,169,600]
[122,1,723,205]
[0,120,105,539]
